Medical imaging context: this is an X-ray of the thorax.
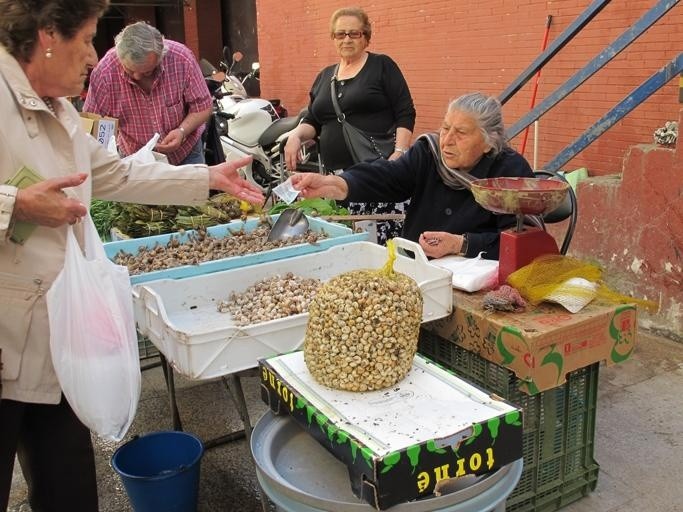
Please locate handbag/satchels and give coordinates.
[331,63,397,167]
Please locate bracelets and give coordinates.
[177,126,187,145]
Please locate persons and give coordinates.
[81,21,215,166]
[284,6,417,171]
[0,0,266,511]
[290,91,538,260]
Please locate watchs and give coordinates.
[394,146,409,155]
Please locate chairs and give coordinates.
[525,171,578,255]
[279,135,335,183]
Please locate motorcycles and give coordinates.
[192,45,326,193]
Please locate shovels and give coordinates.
[267,207,309,241]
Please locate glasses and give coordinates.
[333,31,362,39]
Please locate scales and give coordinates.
[470,177,570,292]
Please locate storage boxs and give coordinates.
[100,213,368,284]
[257,350,526,511]
[132,238,453,381]
[421,288,639,395]
